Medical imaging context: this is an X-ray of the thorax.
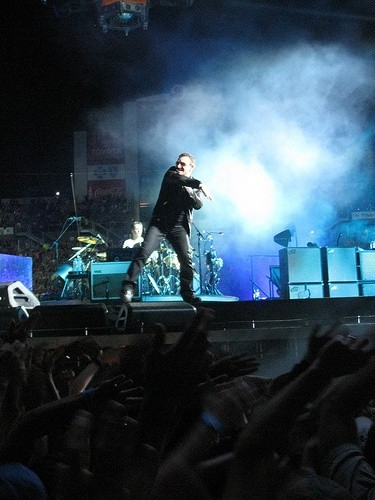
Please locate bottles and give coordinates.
[72,255,87,272]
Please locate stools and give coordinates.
[57,271,93,301]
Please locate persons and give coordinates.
[120,153,211,303]
[122,221,145,249]
[0,308,375,500]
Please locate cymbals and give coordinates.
[72,247,98,252]
[76,236,103,244]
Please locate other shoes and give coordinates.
[186,297,201,305]
[120,286,134,304]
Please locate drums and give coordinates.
[163,253,180,271]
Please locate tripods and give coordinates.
[146,222,224,296]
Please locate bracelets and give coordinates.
[82,388,94,405]
[201,411,223,433]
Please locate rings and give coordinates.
[346,334,356,341]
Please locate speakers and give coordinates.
[357,248,375,298]
[90,261,142,301]
[278,247,324,300]
[0,280,40,309]
[320,247,360,298]
